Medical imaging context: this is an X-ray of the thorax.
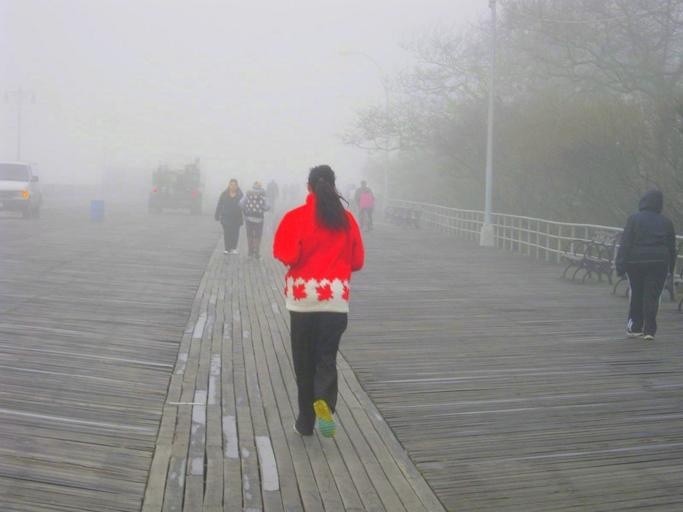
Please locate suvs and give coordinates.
[0,162,42,217]
[149,168,203,214]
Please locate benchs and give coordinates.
[562,238,674,302]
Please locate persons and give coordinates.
[352,180,375,228]
[614,189,678,340]
[268,166,367,439]
[214,178,243,254]
[236,180,270,260]
[264,180,279,210]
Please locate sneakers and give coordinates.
[624,327,655,341]
[224,249,261,260]
[293,399,337,438]
